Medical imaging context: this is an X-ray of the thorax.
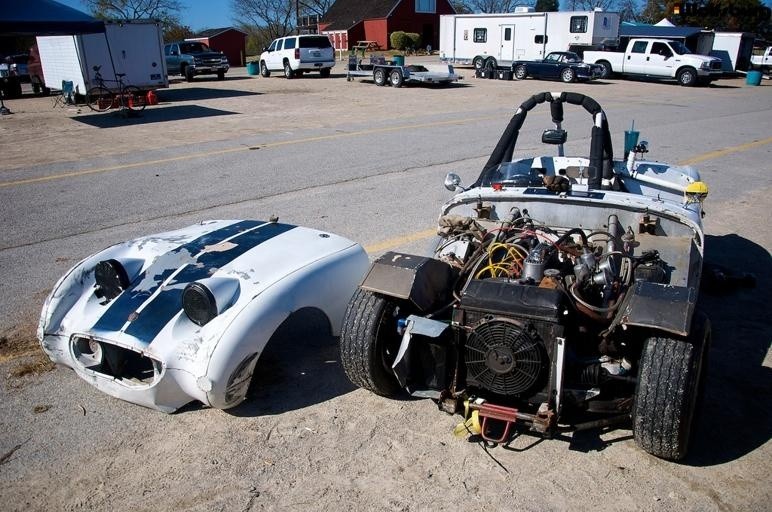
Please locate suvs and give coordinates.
[335,79,726,459]
[4,52,34,81]
[164,40,230,81]
[0,57,25,98]
[257,34,337,78]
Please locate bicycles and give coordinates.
[85,70,150,113]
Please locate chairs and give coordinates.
[48,79,80,108]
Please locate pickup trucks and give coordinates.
[583,36,725,86]
[747,44,772,69]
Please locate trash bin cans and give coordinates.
[0,76,22,100]
[246,61,259,75]
[746,67,763,85]
[393,55,404,66]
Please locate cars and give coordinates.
[511,49,608,85]
